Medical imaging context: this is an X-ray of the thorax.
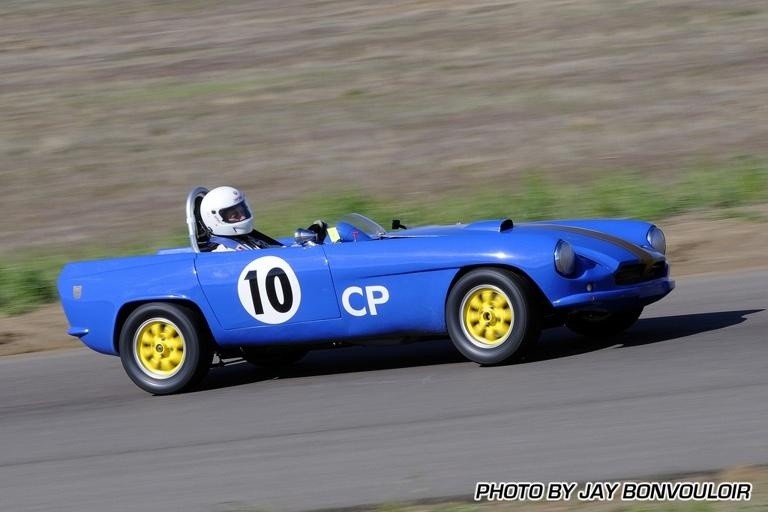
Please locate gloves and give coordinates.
[313,220,328,229]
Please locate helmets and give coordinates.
[200,186,253,236]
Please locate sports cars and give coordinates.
[56,185,680,396]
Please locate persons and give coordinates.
[198,187,327,252]
